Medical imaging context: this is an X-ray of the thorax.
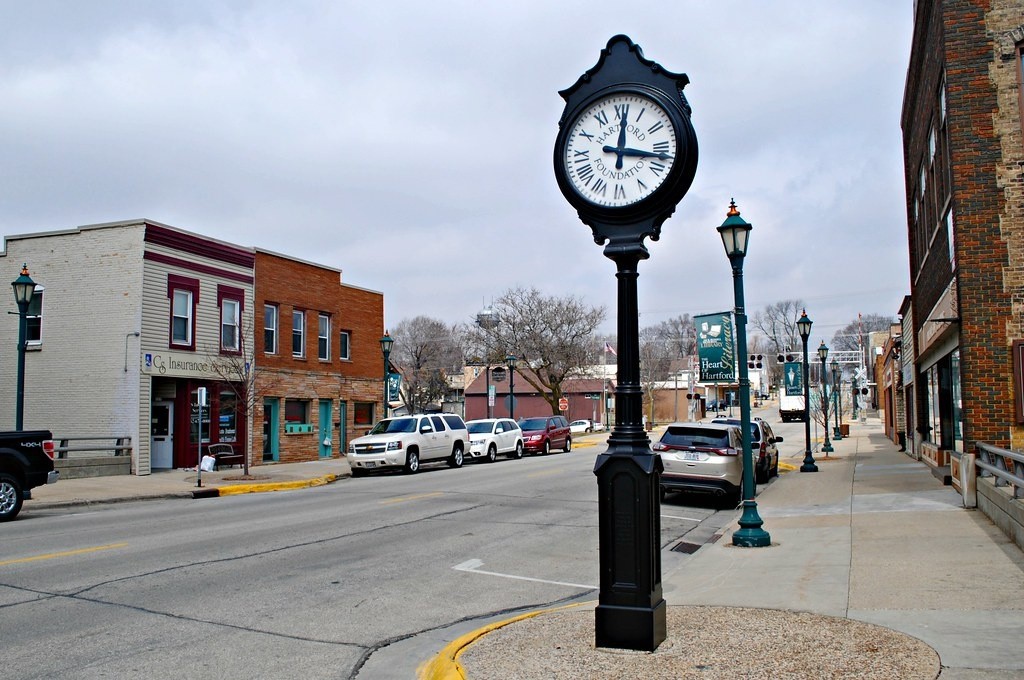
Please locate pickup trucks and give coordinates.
[0,426,59,522]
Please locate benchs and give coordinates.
[208,443,244,471]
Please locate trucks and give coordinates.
[779,384,818,422]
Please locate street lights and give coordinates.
[506,352,518,419]
[10,262,37,431]
[716,192,777,550]
[378,330,395,420]
[850,376,859,421]
[796,307,820,472]
[835,368,847,439]
[816,339,834,453]
[829,358,844,442]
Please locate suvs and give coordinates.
[711,415,784,484]
[347,412,471,474]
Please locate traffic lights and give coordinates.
[861,389,868,395]
[749,362,755,370]
[757,354,763,360]
[694,394,701,399]
[756,362,763,369]
[776,355,785,363]
[786,354,794,362]
[686,393,692,399]
[854,388,859,394]
[749,355,755,361]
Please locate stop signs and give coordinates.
[559,398,568,411]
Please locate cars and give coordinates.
[463,417,526,462]
[569,419,605,432]
[705,398,728,412]
[514,415,573,457]
[650,421,761,506]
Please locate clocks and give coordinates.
[563,86,677,208]
